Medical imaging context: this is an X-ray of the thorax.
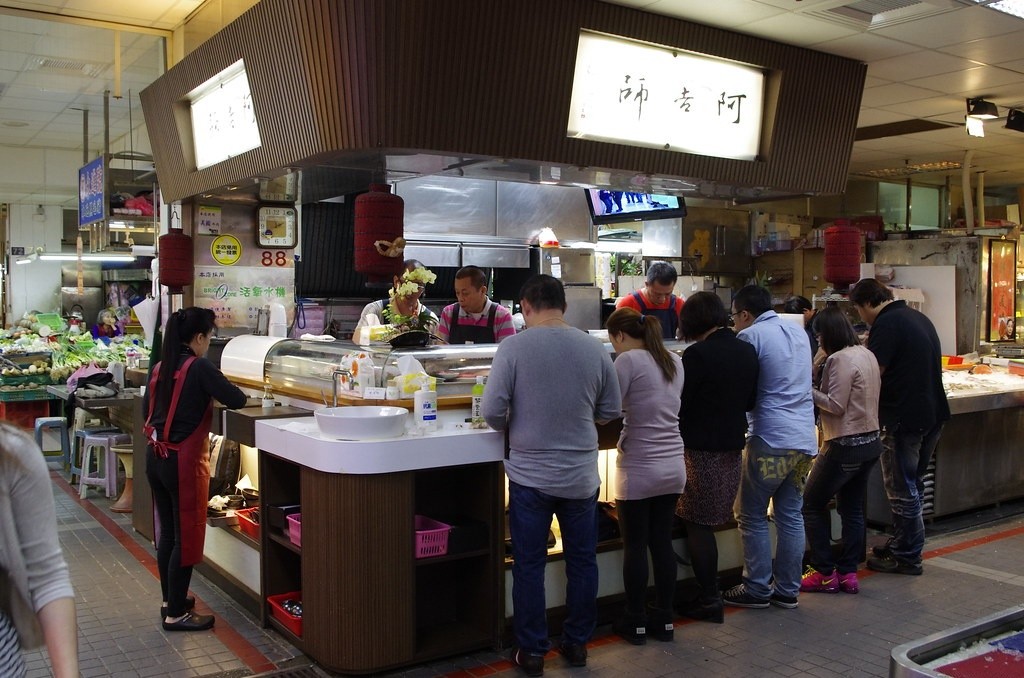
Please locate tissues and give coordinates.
[393,355,437,399]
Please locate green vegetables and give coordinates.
[0,338,14,347]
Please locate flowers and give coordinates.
[382,267,448,347]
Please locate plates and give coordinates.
[436,372,459,379]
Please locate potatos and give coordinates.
[1,358,51,376]
[14,330,32,338]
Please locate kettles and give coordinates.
[66,304,85,321]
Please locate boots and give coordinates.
[645,606,674,641]
[678,573,724,623]
[612,607,646,644]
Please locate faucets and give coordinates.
[321,370,355,408]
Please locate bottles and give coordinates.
[262,385,275,408]
[472,376,488,429]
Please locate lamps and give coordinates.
[966,98,998,119]
[1005,110,1024,134]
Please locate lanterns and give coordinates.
[354,184,405,282]
[824,220,861,290]
[159,228,194,294]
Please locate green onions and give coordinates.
[63,344,121,367]
[50,350,69,381]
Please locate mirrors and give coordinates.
[988,239,1016,342]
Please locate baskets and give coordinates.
[266,592,304,637]
[286,513,301,547]
[414,515,452,558]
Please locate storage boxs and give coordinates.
[757,213,814,241]
[0,400,50,429]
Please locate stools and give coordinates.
[35,417,129,500]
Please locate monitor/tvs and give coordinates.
[584,189,688,226]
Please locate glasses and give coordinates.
[731,309,749,320]
[814,333,823,341]
[398,277,424,291]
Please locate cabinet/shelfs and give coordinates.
[812,289,924,349]
[202,397,508,678]
[78,154,156,231]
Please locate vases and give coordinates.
[389,331,429,347]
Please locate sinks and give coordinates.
[314,405,410,440]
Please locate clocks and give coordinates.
[256,205,298,249]
[258,171,301,202]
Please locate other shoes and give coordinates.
[162,610,215,631]
[161,596,195,618]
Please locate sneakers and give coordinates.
[833,567,859,593]
[511,646,544,676]
[867,554,922,575]
[559,639,587,666]
[768,581,798,609]
[873,536,895,558]
[799,565,840,593]
[722,583,770,608]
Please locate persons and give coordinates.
[850,278,951,575]
[801,305,881,594]
[600,190,614,213]
[142,306,247,634]
[352,259,516,345]
[611,191,623,212]
[616,261,685,339]
[479,274,623,678]
[606,307,686,645]
[719,286,816,608]
[91,309,121,340]
[646,194,652,203]
[1000,318,1014,340]
[0,418,80,678]
[782,295,827,391]
[636,193,643,203]
[678,292,759,624]
[625,192,635,203]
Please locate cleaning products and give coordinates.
[412,376,438,431]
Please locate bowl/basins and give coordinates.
[208,477,261,515]
